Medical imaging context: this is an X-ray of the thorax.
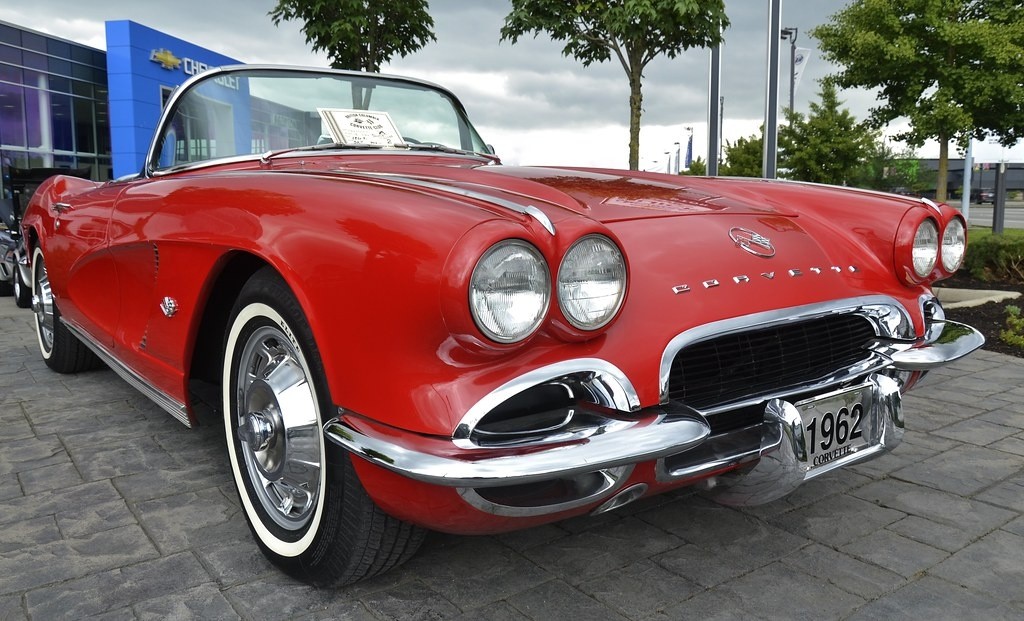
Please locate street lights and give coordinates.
[649,125,694,175]
[781,27,797,128]
[720,95,723,162]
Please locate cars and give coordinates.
[1,164,93,307]
[960,188,995,205]
[19,61,989,590]
